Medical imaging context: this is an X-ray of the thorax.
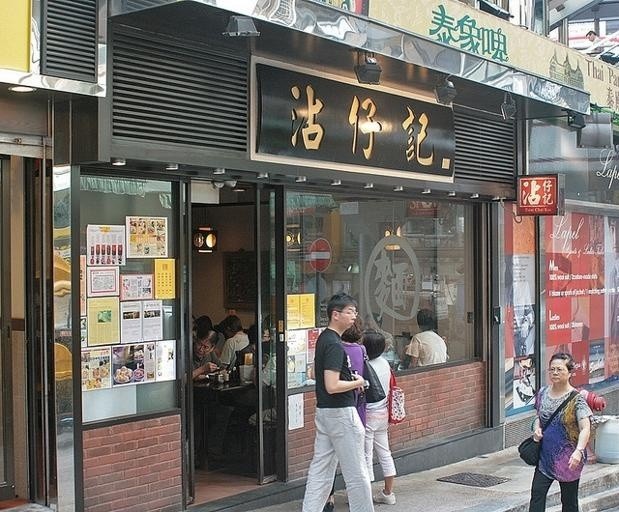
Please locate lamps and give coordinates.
[562,107,586,129]
[433,72,457,105]
[353,48,382,86]
[193,206,218,253]
[287,208,300,252]
[385,206,401,250]
[500,91,518,120]
[222,14,261,40]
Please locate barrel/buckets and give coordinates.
[594,419,619,464]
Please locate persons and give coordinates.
[322,323,369,511]
[191,311,278,474]
[361,332,398,506]
[363,312,397,372]
[404,307,447,370]
[585,29,619,65]
[300,292,376,512]
[529,352,595,512]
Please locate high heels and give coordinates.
[324,502,334,512]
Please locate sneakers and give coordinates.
[373,489,396,505]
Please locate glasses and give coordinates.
[339,310,360,315]
[196,342,214,352]
[547,366,566,373]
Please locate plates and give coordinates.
[113,367,134,384]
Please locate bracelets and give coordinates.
[576,448,584,456]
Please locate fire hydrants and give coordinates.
[577,386,606,464]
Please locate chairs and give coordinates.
[392,331,412,357]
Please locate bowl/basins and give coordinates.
[133,368,144,381]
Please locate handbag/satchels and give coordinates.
[518,435,540,466]
[361,360,386,403]
[388,386,405,423]
